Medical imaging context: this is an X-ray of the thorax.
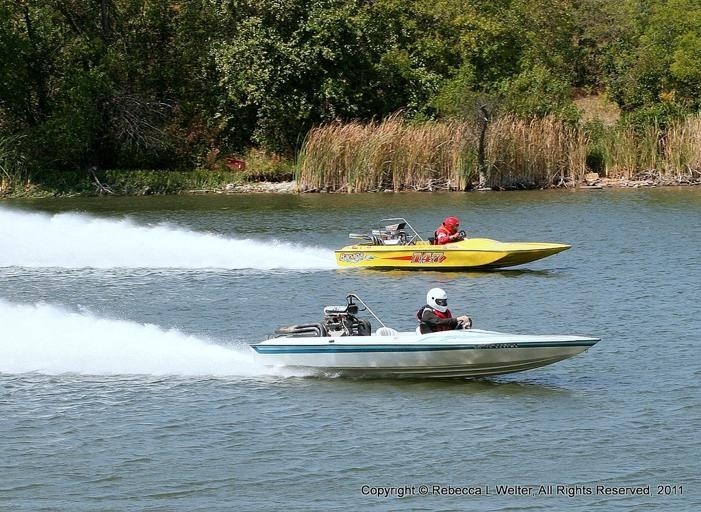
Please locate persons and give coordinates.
[416,287,470,334]
[433,217,459,244]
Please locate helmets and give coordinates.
[427,287,449,314]
[443,216,460,234]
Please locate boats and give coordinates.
[333,216,572,270]
[246,287,602,379]
